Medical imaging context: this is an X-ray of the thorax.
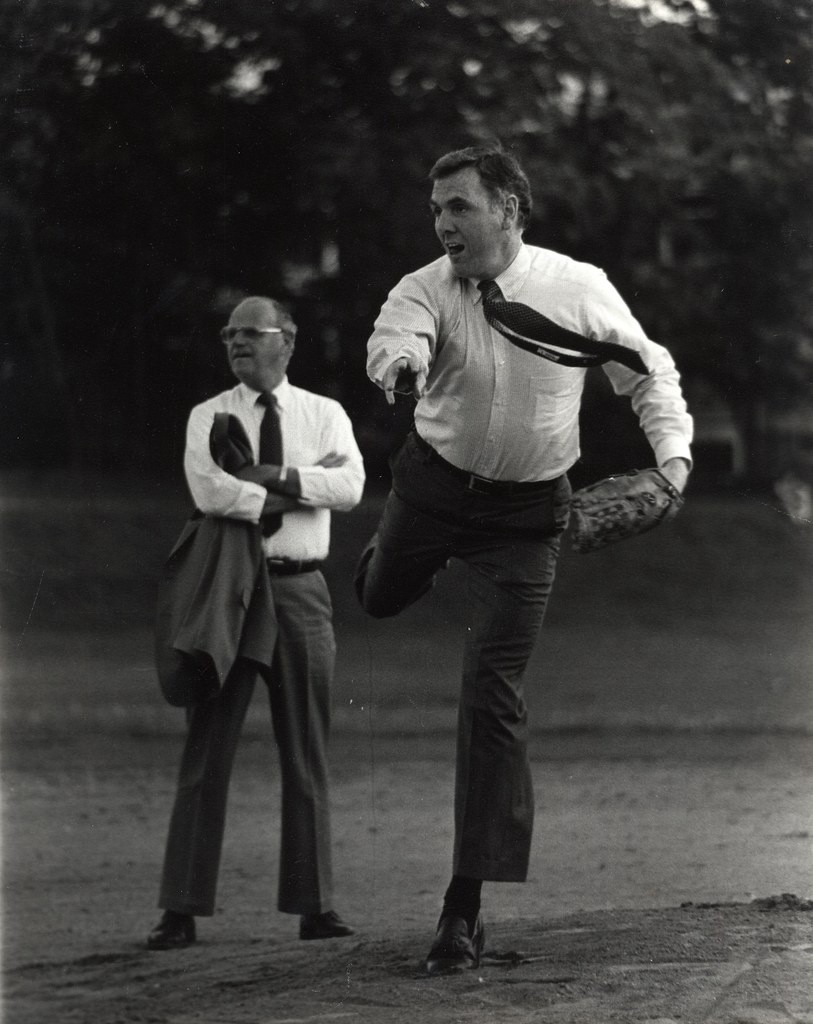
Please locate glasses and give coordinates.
[218,324,284,346]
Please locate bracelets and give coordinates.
[277,465,287,489]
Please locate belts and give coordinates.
[410,433,564,498]
[265,559,320,578]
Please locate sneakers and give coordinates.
[298,909,357,939]
[146,915,197,950]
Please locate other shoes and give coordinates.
[424,918,486,972]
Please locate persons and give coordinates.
[145,296,368,949]
[354,145,695,977]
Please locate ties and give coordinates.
[255,394,284,538]
[477,281,652,375]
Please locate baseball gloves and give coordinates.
[569,465,689,554]
